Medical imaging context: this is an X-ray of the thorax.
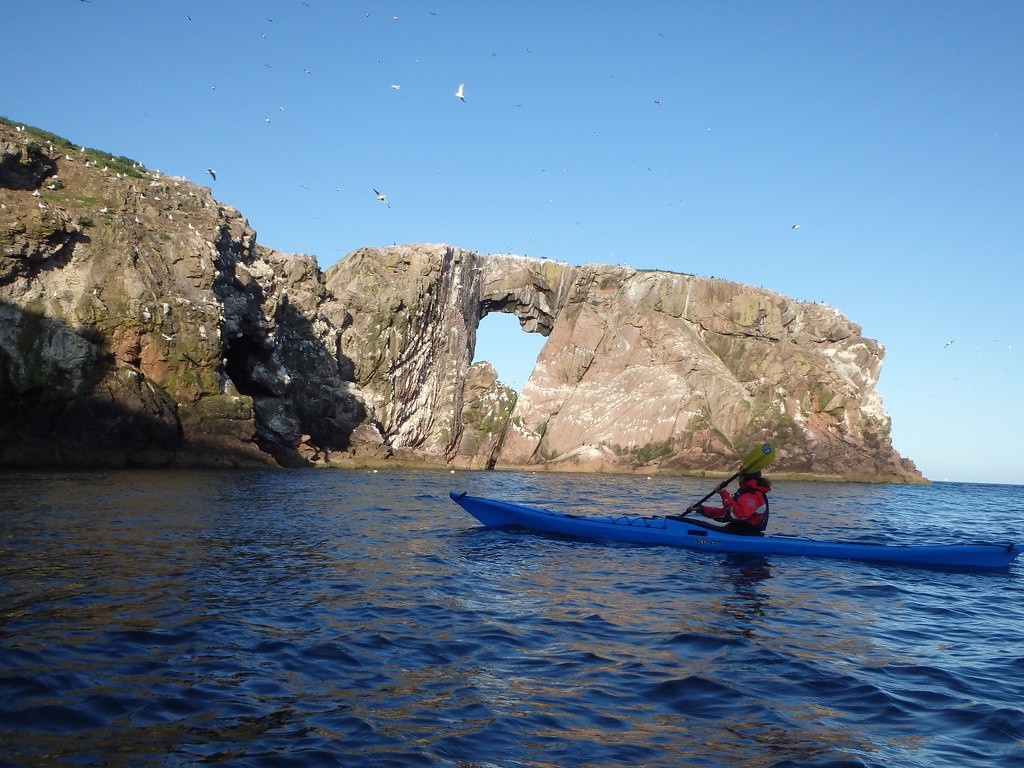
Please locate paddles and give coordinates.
[679,442,776,517]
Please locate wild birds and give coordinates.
[943,339,955,348]
[79,0,799,279]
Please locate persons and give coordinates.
[687,466,772,531]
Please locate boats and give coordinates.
[448,489,1024,570]
